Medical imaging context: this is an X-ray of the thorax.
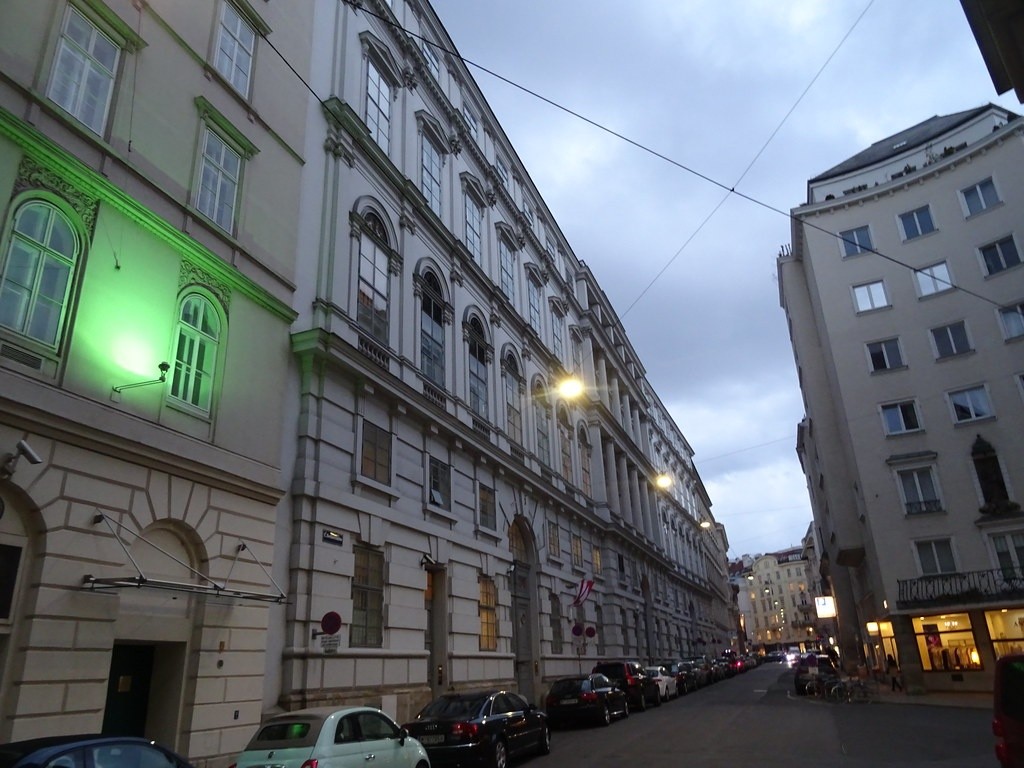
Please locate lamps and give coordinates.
[112,360,170,393]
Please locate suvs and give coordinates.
[592,661,663,710]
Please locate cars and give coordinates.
[228,706,431,768]
[643,666,680,701]
[402,692,551,768]
[661,646,801,698]
[0,733,191,768]
[795,654,834,698]
[546,672,629,727]
[806,649,821,657]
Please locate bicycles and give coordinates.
[806,671,877,706]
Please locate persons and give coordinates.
[886,653,903,692]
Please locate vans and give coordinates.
[991,653,1024,768]
